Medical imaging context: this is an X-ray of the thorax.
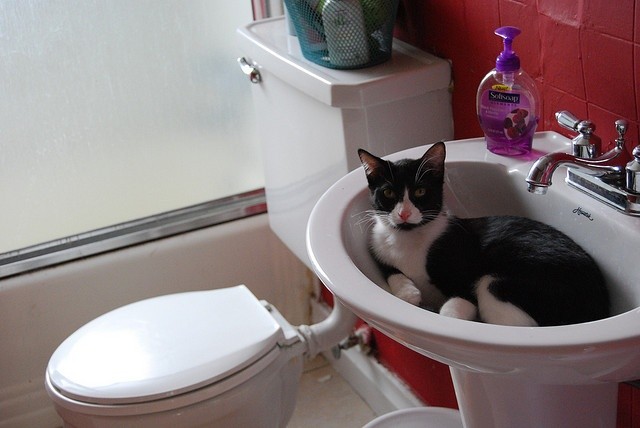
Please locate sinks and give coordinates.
[307,129,639,385]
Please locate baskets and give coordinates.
[284,0,401,69]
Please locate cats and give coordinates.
[347,140,613,328]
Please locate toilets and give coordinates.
[44,15,455,426]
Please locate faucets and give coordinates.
[526,118,635,198]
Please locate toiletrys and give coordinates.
[475,25,539,156]
[323,1,369,66]
